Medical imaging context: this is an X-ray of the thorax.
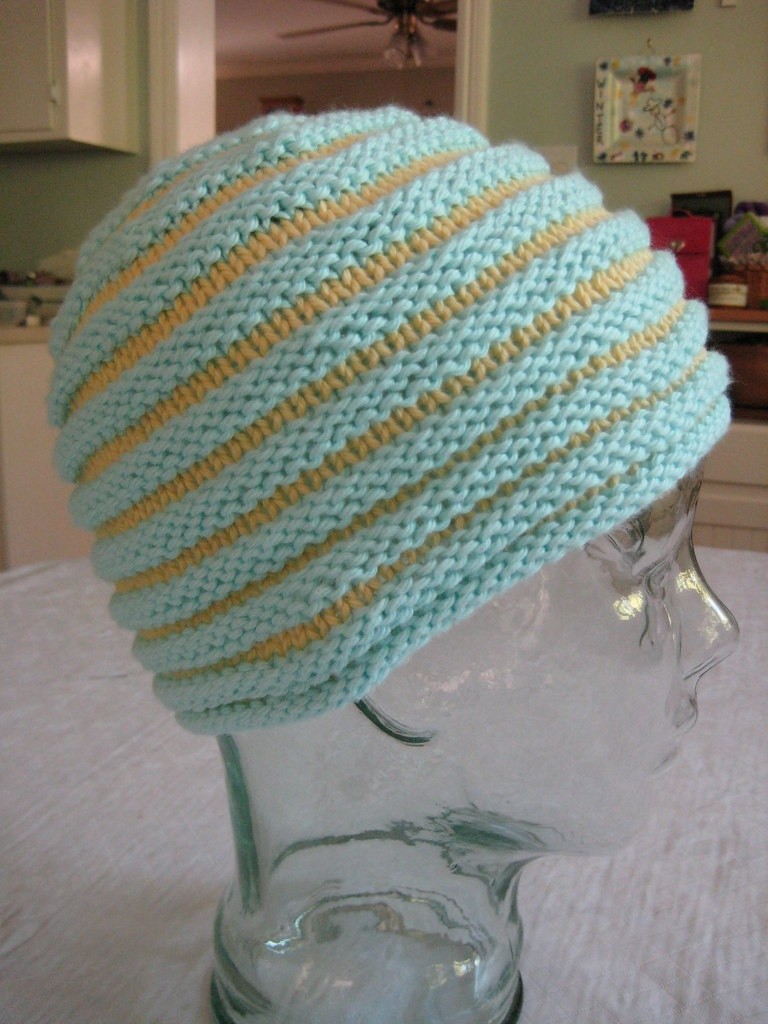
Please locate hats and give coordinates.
[48,104,732,736]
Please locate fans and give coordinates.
[278,0,457,42]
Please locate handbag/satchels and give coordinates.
[669,190,732,245]
[644,207,715,306]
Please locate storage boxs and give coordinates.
[710,341,767,410]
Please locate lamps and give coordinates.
[382,7,432,73]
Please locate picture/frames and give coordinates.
[591,55,701,164]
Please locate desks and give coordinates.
[0,530,768,1023]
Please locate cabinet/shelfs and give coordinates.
[690,310,768,553]
[0,0,139,156]
